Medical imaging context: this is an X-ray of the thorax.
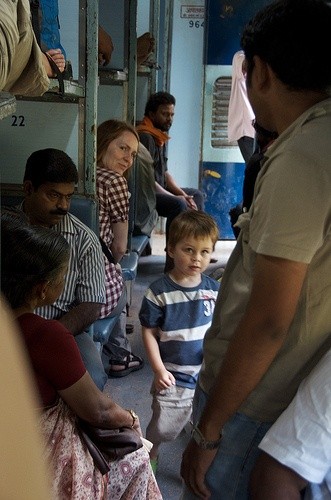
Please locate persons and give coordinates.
[145,211,225,479]
[0,0,331,500]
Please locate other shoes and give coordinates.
[150,459,158,475]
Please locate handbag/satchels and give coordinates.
[77,421,143,474]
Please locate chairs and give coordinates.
[131,235,148,255]
[68,196,115,342]
[117,252,139,277]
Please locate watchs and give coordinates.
[189,428,223,449]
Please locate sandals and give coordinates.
[103,343,130,358]
[108,354,144,379]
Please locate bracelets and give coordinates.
[126,409,138,429]
